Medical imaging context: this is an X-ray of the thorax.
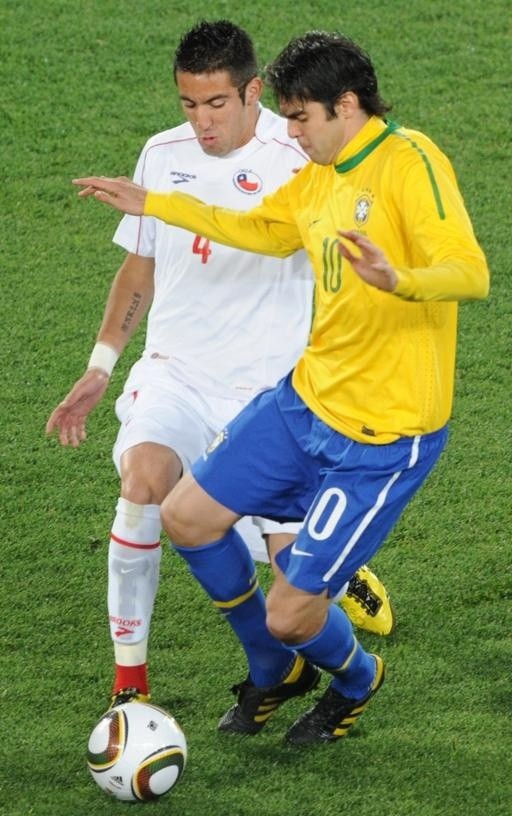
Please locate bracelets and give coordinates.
[85,342,121,378]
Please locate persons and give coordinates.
[42,15,394,710]
[71,30,490,748]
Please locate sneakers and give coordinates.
[216,649,323,739]
[100,682,152,713]
[338,563,401,638]
[282,647,388,747]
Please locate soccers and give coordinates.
[85,703,187,803]
